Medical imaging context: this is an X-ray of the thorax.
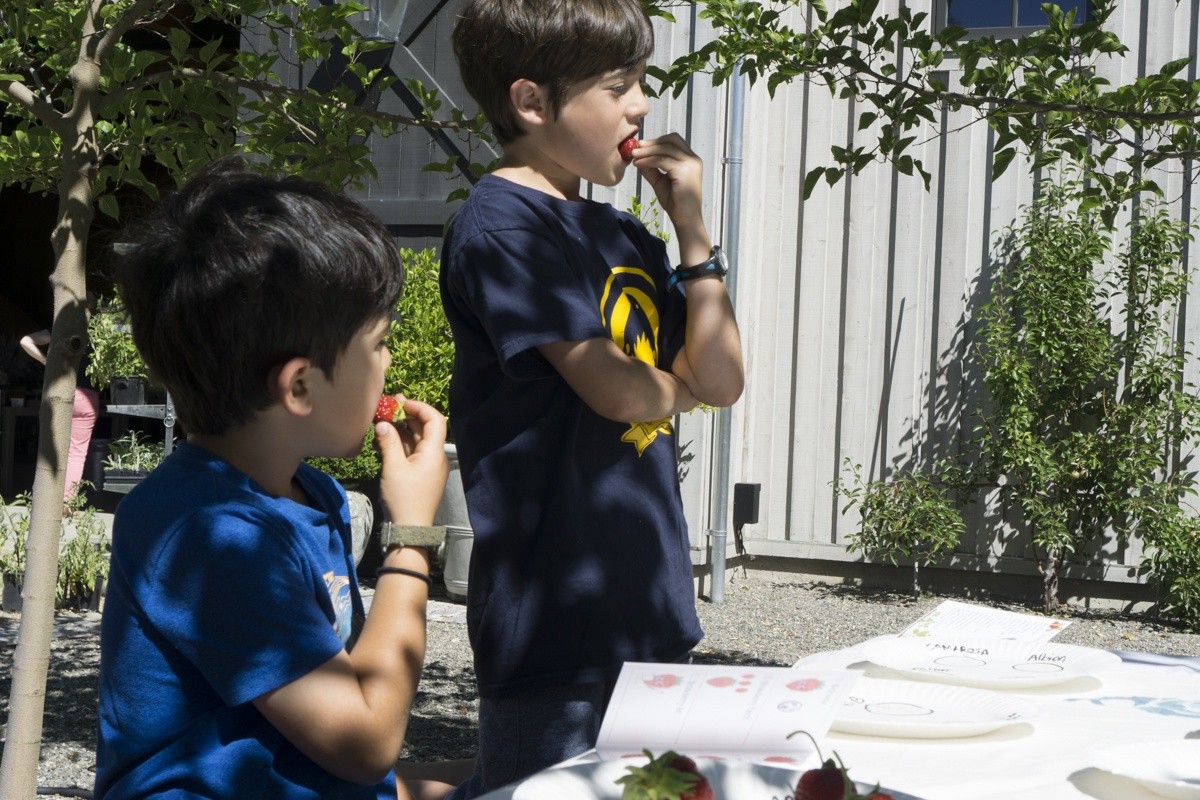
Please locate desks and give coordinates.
[474,641,1200,800]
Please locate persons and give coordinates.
[19,291,102,501]
[438,0,744,797]
[94,161,480,800]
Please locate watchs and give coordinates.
[674,244,730,283]
[376,521,450,568]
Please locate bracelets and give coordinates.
[373,565,432,588]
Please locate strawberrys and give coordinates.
[617,748,891,800]
[618,136,640,161]
[372,396,410,427]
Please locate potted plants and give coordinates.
[86,318,149,405]
[381,246,479,602]
[0,480,111,612]
[304,421,383,575]
